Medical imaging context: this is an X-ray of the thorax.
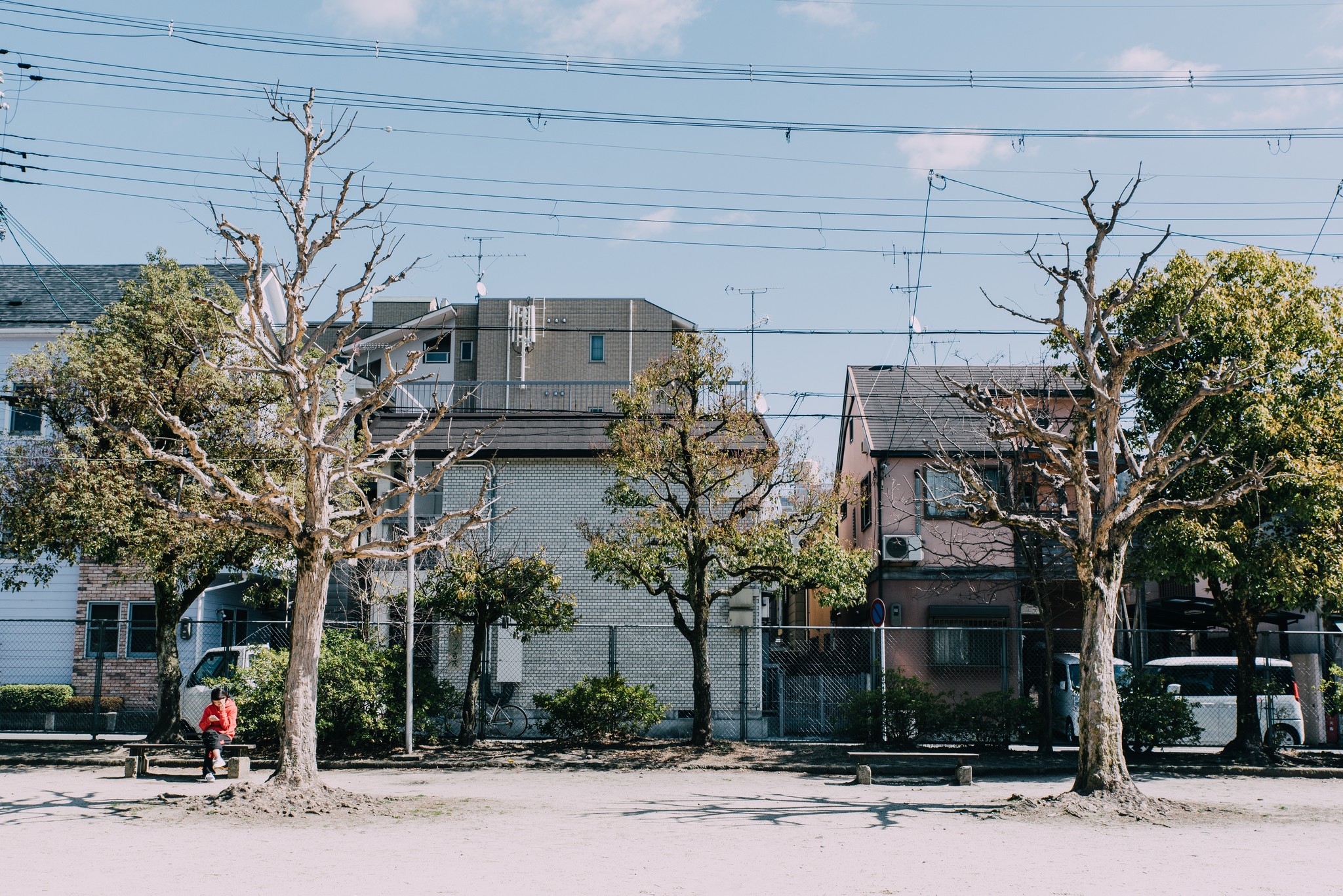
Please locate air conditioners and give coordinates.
[881,535,924,562]
[762,597,770,618]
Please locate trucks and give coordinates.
[180,641,271,738]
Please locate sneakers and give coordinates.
[205,773,215,782]
[212,757,226,770]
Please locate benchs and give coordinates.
[847,752,979,786]
[123,743,256,779]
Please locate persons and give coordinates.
[198,688,238,782]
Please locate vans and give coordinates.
[1053,652,1137,742]
[1111,654,1306,759]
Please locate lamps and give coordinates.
[215,609,227,618]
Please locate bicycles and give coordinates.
[444,694,529,738]
